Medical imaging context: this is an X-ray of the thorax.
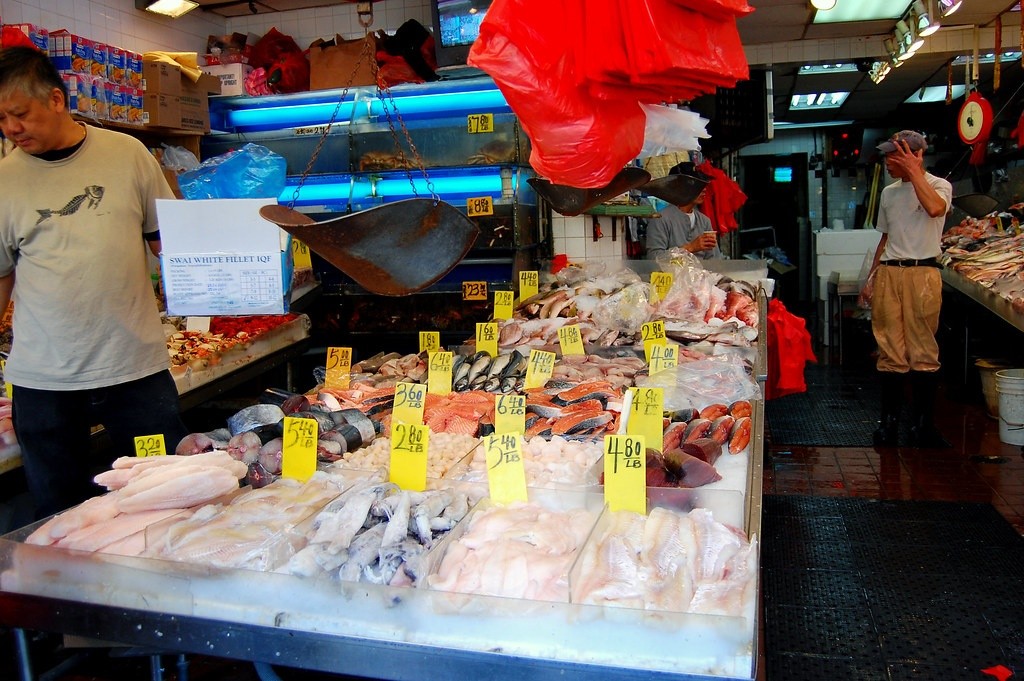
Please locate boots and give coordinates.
[910,368,941,447]
[873,372,905,444]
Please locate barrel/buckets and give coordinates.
[978,367,999,419]
[995,368,1024,446]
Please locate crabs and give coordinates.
[356,151,431,173]
[465,138,530,165]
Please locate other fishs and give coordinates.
[939,217,1024,307]
[516,287,607,318]
[452,350,529,393]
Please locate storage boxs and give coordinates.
[153,193,292,316]
[308,30,377,92]
[4,23,255,133]
[814,226,885,296]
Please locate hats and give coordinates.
[669,162,713,183]
[875,131,929,156]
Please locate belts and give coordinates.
[880,257,944,270]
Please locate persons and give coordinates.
[856,129,952,450]
[0,46,189,515]
[644,161,729,262]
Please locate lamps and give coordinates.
[869,0,961,84]
